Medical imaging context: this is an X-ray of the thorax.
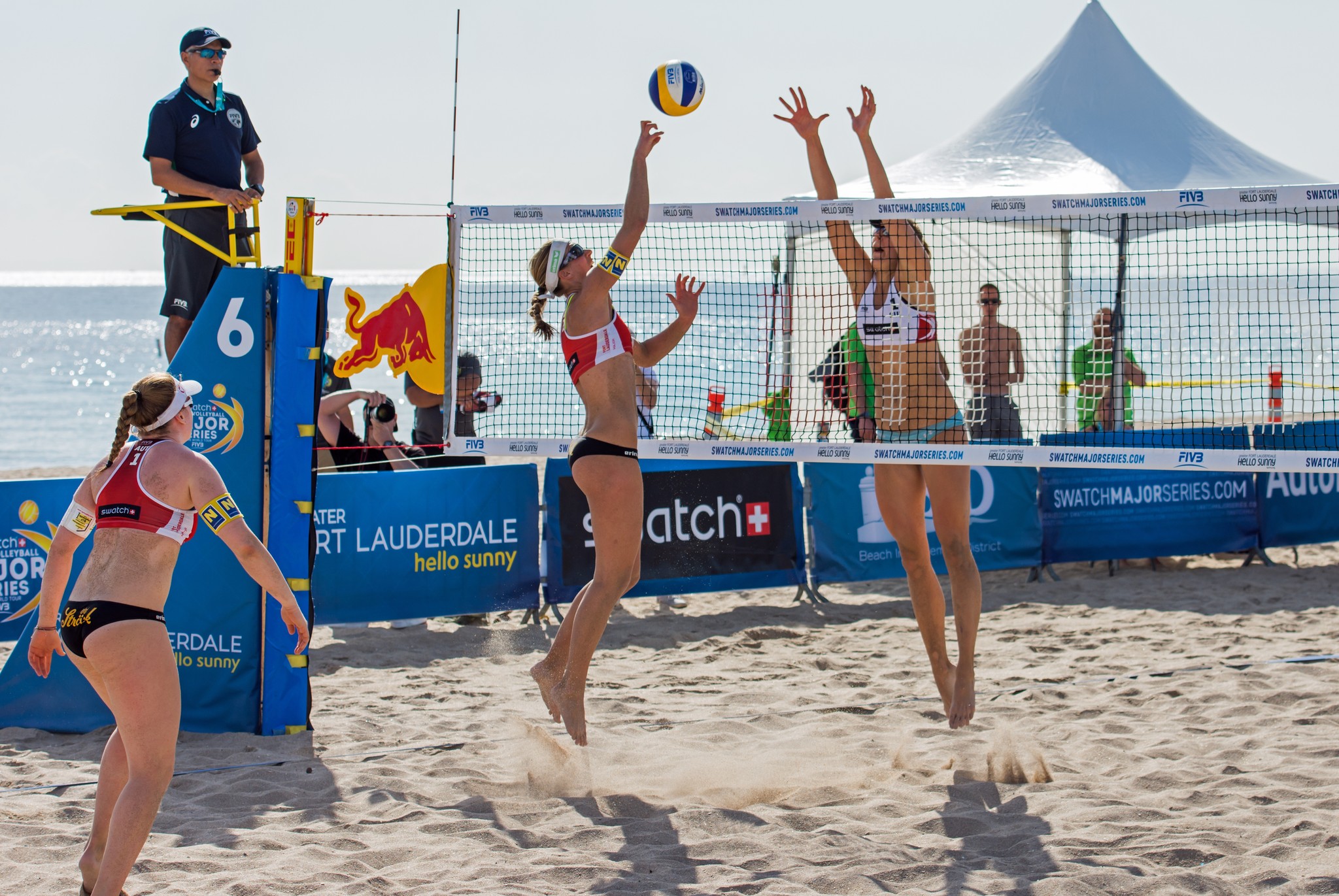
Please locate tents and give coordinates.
[769,0,1339,432]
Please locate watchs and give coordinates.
[250,182,265,197]
[381,441,396,451]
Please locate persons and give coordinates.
[405,352,487,620]
[317,321,354,474]
[959,282,1024,446]
[28,374,311,896]
[142,27,264,366]
[773,84,982,727]
[1074,307,1147,432]
[317,389,429,628]
[805,318,877,443]
[529,120,705,744]
[627,325,688,609]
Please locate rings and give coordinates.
[228,203,232,208]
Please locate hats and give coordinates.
[363,397,398,431]
[180,27,232,53]
[869,220,886,229]
[136,373,203,431]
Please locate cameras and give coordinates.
[367,400,395,421]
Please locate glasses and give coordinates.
[561,244,583,270]
[981,298,998,304]
[873,228,889,236]
[189,49,226,59]
[183,396,193,408]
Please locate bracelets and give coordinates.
[859,409,870,419]
[597,245,632,279]
[199,491,244,535]
[32,623,59,634]
[61,498,97,539]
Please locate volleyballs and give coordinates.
[648,59,706,116]
